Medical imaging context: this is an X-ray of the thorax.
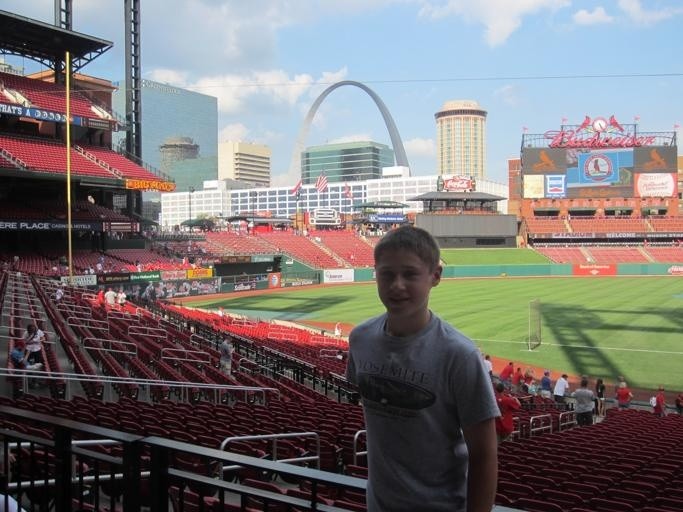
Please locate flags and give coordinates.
[289,181,301,193]
[345,184,353,196]
[315,175,327,192]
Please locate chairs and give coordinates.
[0,71,683,512]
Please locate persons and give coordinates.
[219,335,235,375]
[55,286,63,303]
[346,226,501,512]
[653,386,682,417]
[11,323,45,374]
[10,254,142,277]
[98,287,126,310]
[483,355,633,444]
